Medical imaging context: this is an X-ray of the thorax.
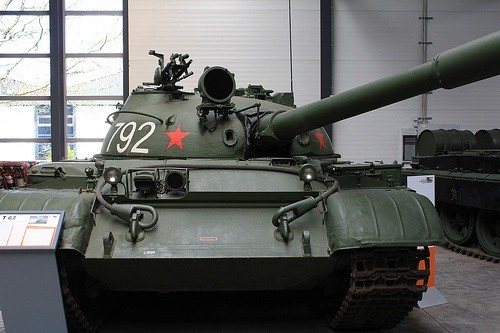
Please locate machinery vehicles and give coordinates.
[1,32,500,332]
[401,128,499,263]
